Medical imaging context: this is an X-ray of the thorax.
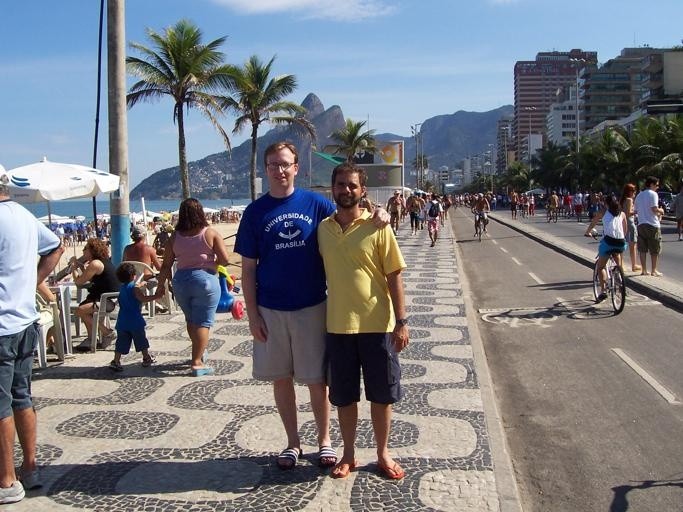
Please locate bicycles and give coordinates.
[545,204,559,226]
[473,211,492,243]
[585,233,628,312]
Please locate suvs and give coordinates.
[657,190,673,210]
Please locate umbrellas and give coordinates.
[1,158,123,234]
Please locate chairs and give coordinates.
[36,261,173,370]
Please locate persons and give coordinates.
[470,193,491,238]
[0,168,64,505]
[315,162,412,481]
[510,187,586,223]
[386,190,469,247]
[233,141,393,470]
[38,198,243,376]
[585,178,682,303]
[485,192,511,210]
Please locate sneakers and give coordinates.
[17,462,43,491]
[100,329,117,349]
[76,338,99,350]
[393,227,398,236]
[598,293,607,300]
[411,224,424,236]
[0,480,25,504]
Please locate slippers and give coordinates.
[332,458,357,478]
[277,448,302,470]
[319,446,337,467]
[378,461,404,479]
[191,349,213,377]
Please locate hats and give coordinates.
[394,190,400,195]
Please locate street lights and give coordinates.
[486,143,495,193]
[499,127,512,196]
[568,58,586,192]
[410,121,425,191]
[524,106,536,190]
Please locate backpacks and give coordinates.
[429,201,439,217]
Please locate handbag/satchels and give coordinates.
[604,235,625,246]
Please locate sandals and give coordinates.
[108,360,123,370]
[142,354,156,366]
